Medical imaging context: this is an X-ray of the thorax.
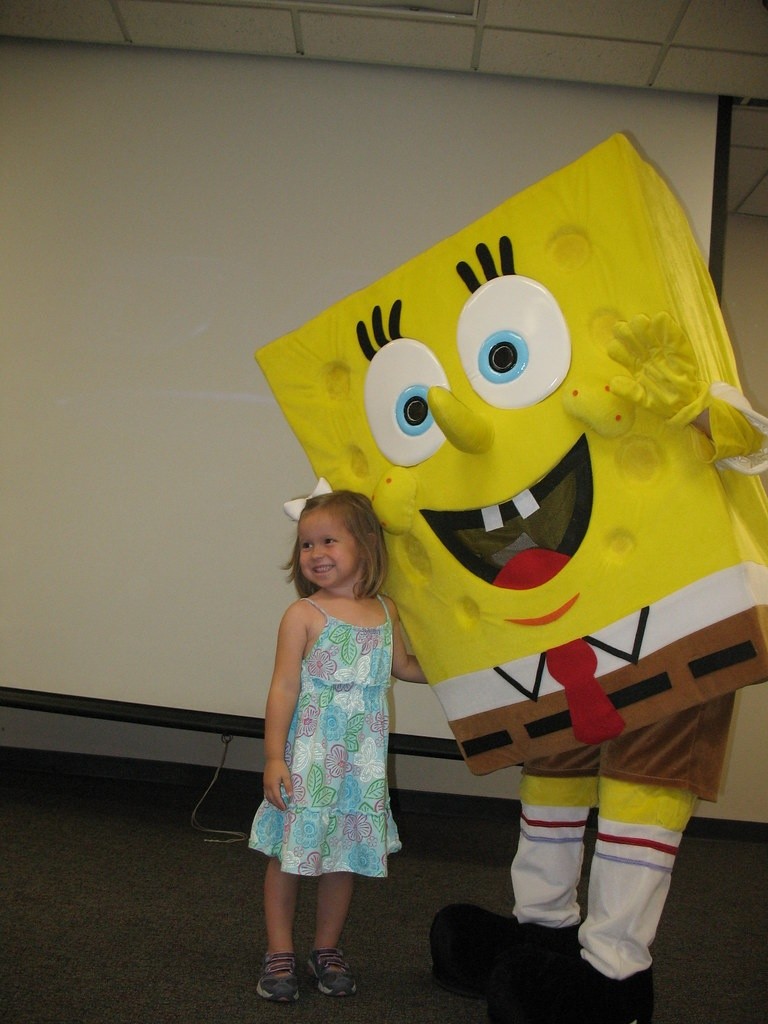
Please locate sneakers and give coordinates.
[306,948,358,997]
[256,951,301,1002]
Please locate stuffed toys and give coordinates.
[254,129,765,1024]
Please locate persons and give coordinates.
[247,482,430,1003]
